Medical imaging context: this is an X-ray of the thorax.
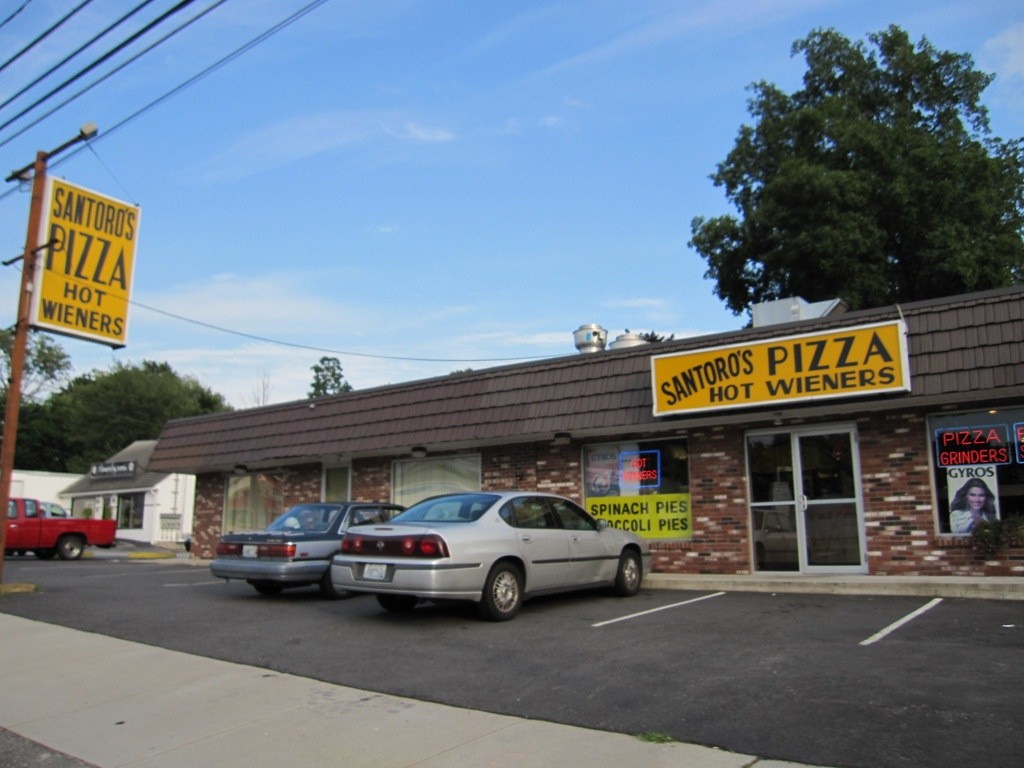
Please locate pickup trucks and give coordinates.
[4,499,116,561]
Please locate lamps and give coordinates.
[234,464,250,476]
[773,412,785,425]
[552,432,575,447]
[410,446,429,459]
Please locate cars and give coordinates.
[331,491,651,621]
[753,509,811,569]
[210,501,410,601]
[8,501,73,556]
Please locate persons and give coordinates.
[950,478,998,533]
